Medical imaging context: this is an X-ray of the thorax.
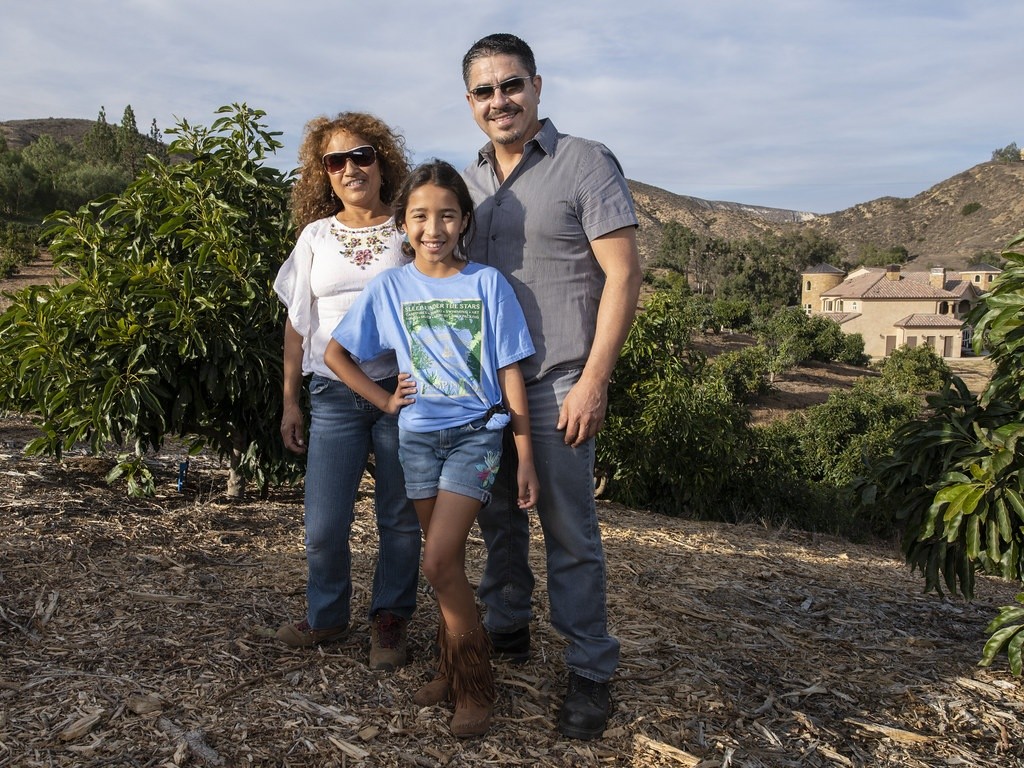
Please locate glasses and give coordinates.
[320,145,378,174]
[470,73,535,102]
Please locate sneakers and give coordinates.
[369,612,408,672]
[276,617,351,647]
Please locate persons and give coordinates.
[458,32,643,740]
[325,161,539,735]
[273,112,409,671]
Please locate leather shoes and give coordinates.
[556,672,613,740]
[486,626,531,662]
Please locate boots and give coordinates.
[443,625,496,738]
[414,611,455,706]
